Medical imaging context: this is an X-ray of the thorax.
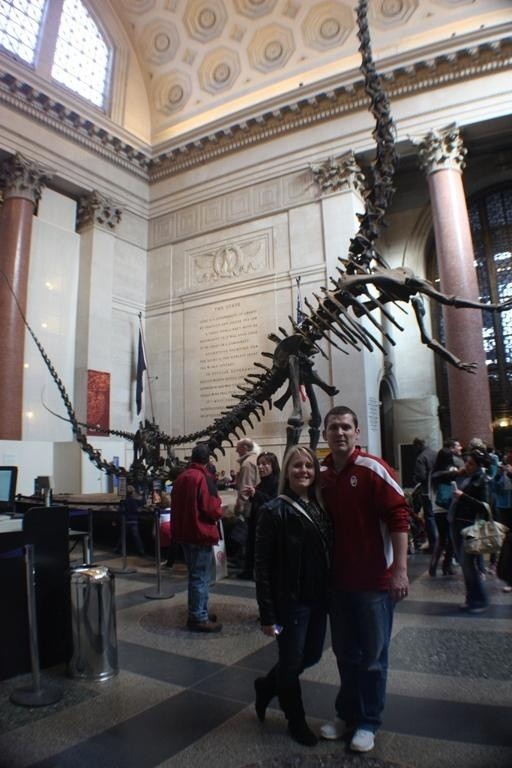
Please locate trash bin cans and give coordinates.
[68,563,120,682]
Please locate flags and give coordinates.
[135,333,146,415]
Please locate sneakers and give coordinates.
[185,612,223,632]
[318,715,357,740]
[426,554,512,615]
[253,674,278,724]
[286,718,317,748]
[349,724,378,753]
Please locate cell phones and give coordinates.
[450,481,458,490]
[275,624,284,635]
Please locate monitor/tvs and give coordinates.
[0,466,18,512]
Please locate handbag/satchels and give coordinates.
[459,500,509,554]
[434,480,455,510]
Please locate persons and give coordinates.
[150,490,173,570]
[238,450,281,559]
[168,443,225,634]
[253,442,332,748]
[403,433,512,616]
[317,404,414,753]
[233,437,262,579]
[111,484,149,559]
